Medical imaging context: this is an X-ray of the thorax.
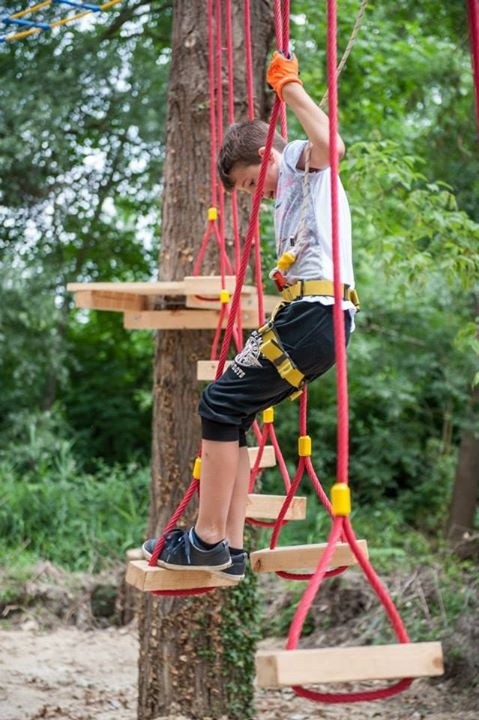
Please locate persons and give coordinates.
[141,51,360,582]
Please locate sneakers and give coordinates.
[208,552,248,581]
[141,528,234,570]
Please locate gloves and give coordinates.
[266,49,303,102]
